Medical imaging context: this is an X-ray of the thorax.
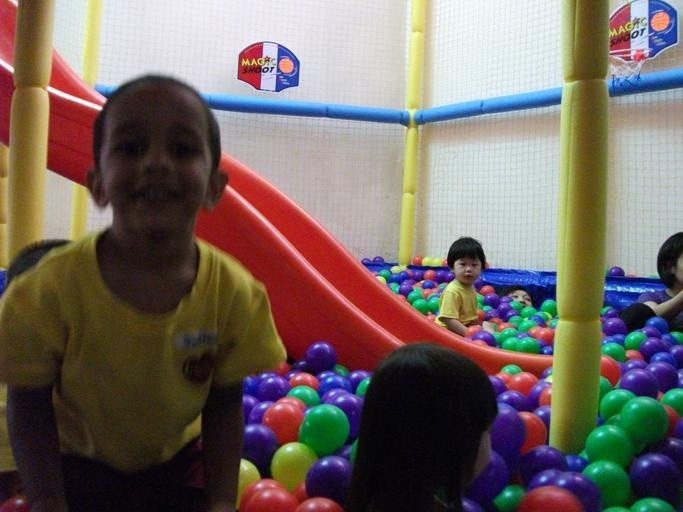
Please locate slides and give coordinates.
[0,0,552,387]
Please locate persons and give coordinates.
[0,73,290,512]
[503,286,533,307]
[346,340,498,511]
[430,236,486,337]
[622,231,682,331]
[0,240,71,472]
